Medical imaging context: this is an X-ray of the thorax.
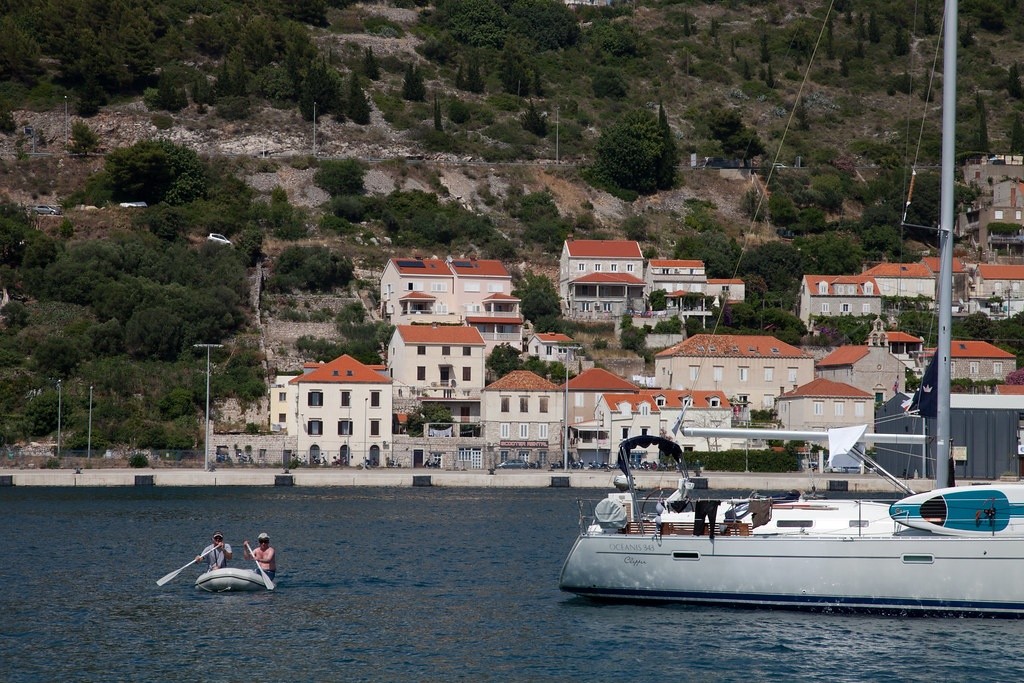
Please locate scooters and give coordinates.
[527,455,680,471]
[333,453,350,467]
[215,450,231,465]
[312,452,330,468]
[362,456,379,467]
[423,457,441,468]
[291,453,307,469]
[237,453,255,465]
[385,457,402,469]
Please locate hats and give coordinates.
[258,533,269,539]
[213,531,224,538]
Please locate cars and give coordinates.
[494,459,531,470]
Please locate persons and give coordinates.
[194,531,233,574]
[243,533,276,582]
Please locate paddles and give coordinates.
[241,537,277,591]
[155,542,224,587]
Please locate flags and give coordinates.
[905,347,938,420]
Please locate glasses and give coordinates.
[259,539,269,544]
[214,537,223,540]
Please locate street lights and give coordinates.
[787,400,792,453]
[193,343,225,470]
[553,344,584,470]
[87,385,93,459]
[742,401,753,472]
[57,378,62,457]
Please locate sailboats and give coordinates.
[548,0,1024,620]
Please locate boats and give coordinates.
[194,567,276,594]
